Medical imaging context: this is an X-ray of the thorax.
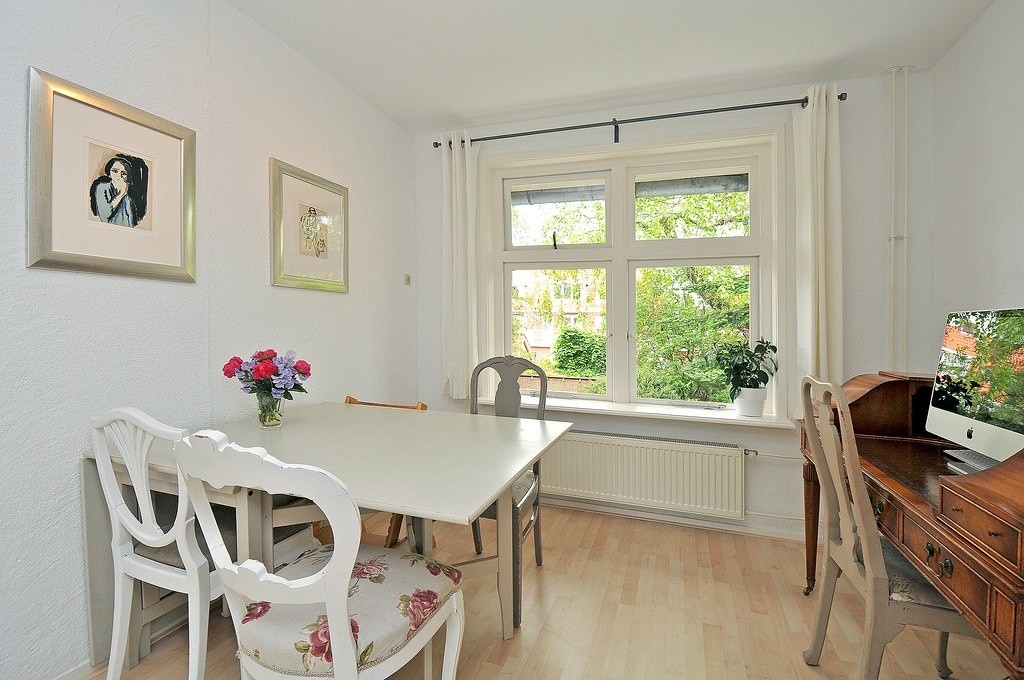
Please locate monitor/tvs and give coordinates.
[925,308,1024,475]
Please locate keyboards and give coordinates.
[943,449,1002,470]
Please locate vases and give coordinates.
[256,392,284,430]
[734,388,767,417]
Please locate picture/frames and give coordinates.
[269,157,350,293]
[25,65,197,283]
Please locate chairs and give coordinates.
[797,374,987,680]
[418,355,548,627]
[88,408,319,680]
[170,429,466,680]
[333,396,436,550]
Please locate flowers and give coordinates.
[705,337,779,402]
[221,347,313,400]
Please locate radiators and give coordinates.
[543,431,747,527]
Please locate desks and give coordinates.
[795,370,1024,680]
[81,401,574,680]
